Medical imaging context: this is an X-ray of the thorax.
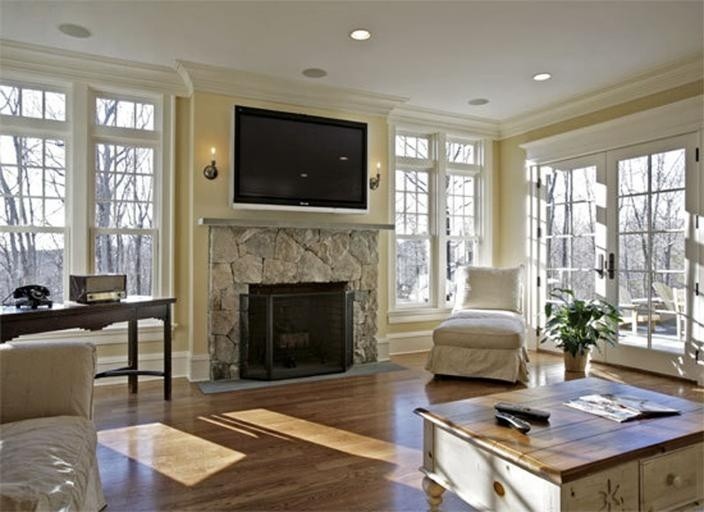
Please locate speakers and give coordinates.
[69,274,127,304]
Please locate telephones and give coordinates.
[13,284,52,309]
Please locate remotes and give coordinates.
[494,401,551,420]
[495,411,531,432]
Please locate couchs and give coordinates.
[0,341,107,512]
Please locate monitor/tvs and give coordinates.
[228,103,370,215]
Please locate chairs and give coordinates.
[618,285,660,334]
[673,287,685,342]
[425,264,530,388]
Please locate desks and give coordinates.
[0,294,177,401]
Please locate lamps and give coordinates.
[370,160,381,190]
[203,145,218,180]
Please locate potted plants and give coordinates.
[535,287,624,372]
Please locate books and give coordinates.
[560,394,643,424]
[599,388,680,416]
